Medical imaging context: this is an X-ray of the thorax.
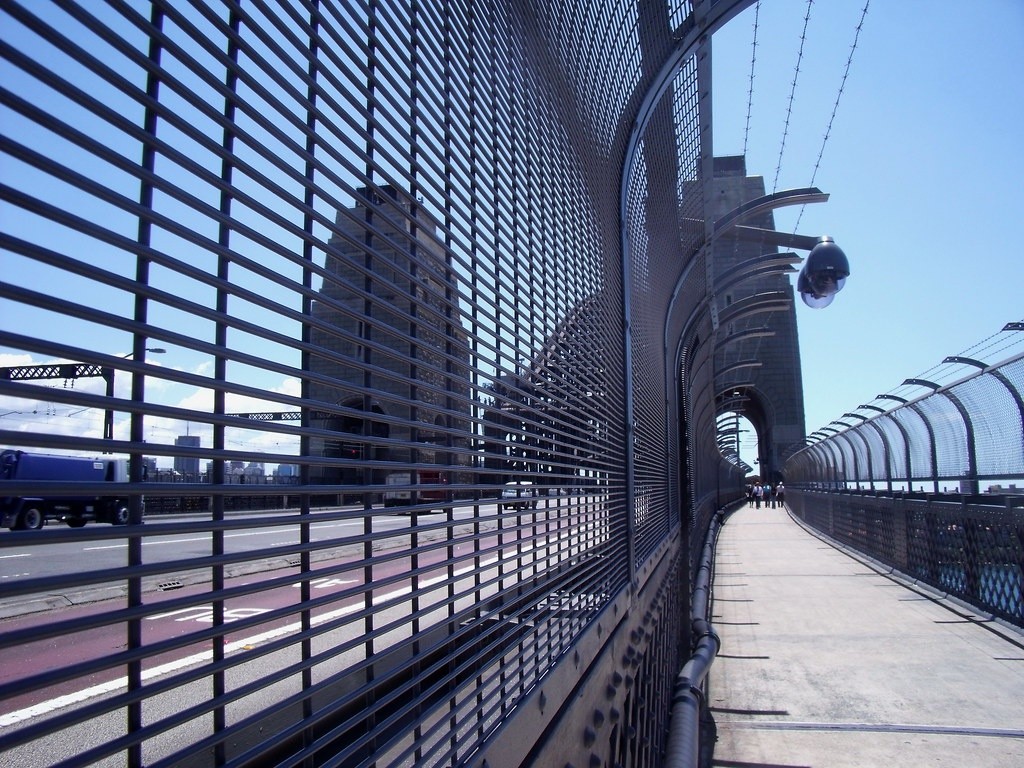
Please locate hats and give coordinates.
[779,481,782,484]
[756,482,760,485]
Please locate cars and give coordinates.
[572,488,586,495]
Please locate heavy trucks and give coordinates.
[0,447,132,531]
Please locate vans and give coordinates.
[500,481,540,510]
[548,488,567,496]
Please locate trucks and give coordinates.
[382,473,422,508]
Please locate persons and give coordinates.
[775,482,785,508]
[748,482,776,509]
